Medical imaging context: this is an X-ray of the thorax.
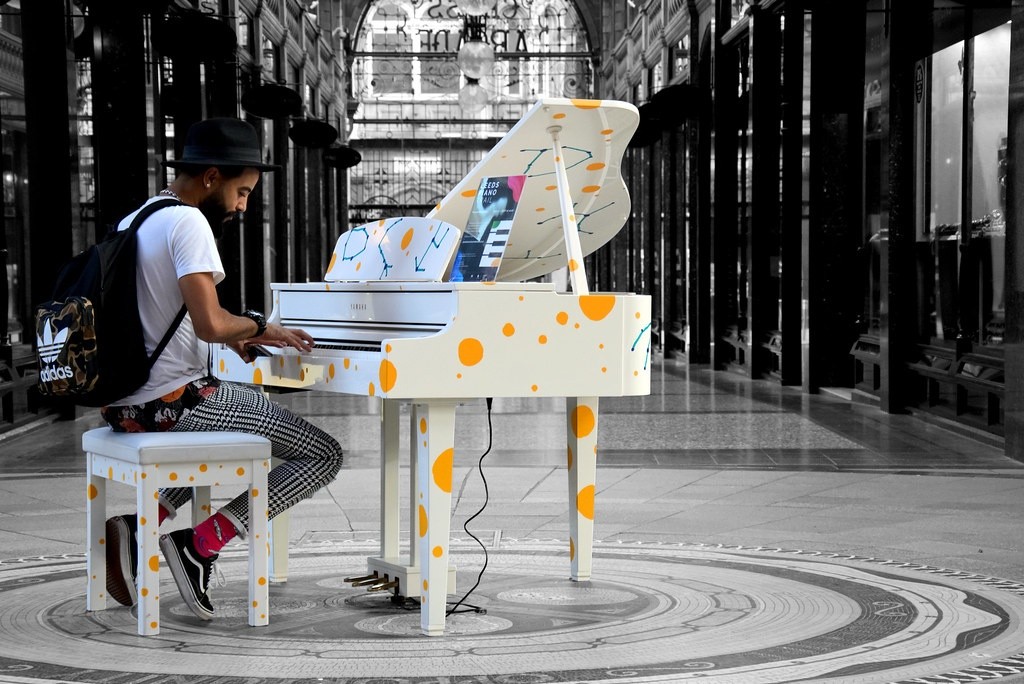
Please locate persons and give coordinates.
[101,117,344,620]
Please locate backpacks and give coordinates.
[34,200,191,407]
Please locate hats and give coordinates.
[160,118,282,173]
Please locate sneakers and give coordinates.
[160,528,222,621]
[106,513,141,606]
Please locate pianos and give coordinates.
[209,96,653,637]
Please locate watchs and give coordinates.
[241,309,267,338]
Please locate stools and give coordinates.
[83,425,273,635]
